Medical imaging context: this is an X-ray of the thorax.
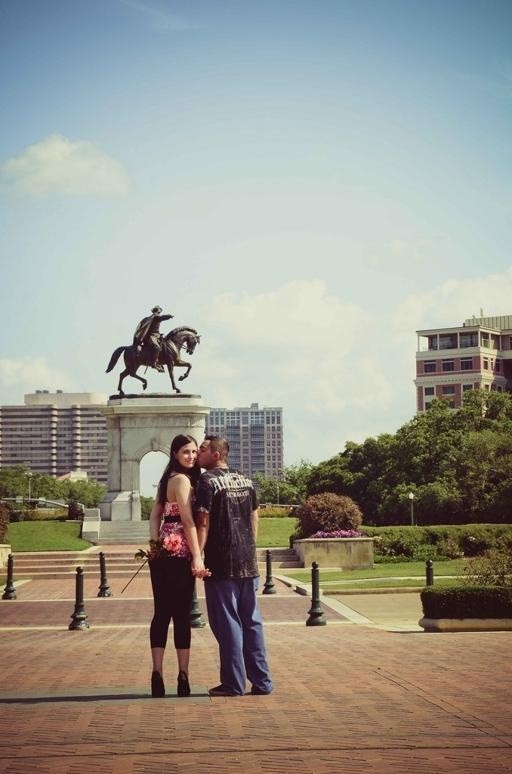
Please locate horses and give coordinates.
[106,326,202,394]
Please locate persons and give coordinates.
[147,433,213,698]
[135,304,176,370]
[190,434,273,697]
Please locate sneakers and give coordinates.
[208,686,241,696]
[244,686,269,695]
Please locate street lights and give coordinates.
[409,490,416,525]
[23,470,35,502]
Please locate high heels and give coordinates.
[177,671,190,697]
[151,671,165,698]
